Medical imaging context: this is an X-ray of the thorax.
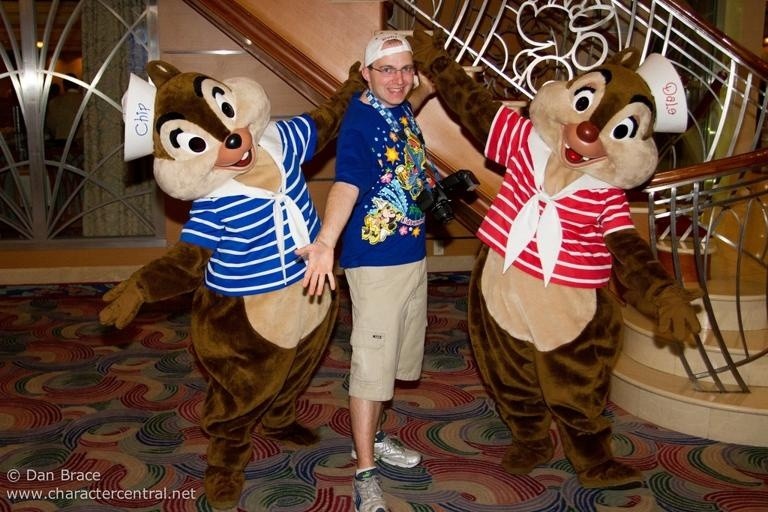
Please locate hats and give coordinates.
[364,33,413,66]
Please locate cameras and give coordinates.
[418,169,481,226]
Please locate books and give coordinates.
[403,29,708,490]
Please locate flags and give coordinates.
[97,60,370,509]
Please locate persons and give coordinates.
[294,31,432,511]
[46,72,84,225]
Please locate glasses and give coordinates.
[371,64,413,75]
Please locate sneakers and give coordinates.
[350,431,423,469]
[352,468,389,512]
